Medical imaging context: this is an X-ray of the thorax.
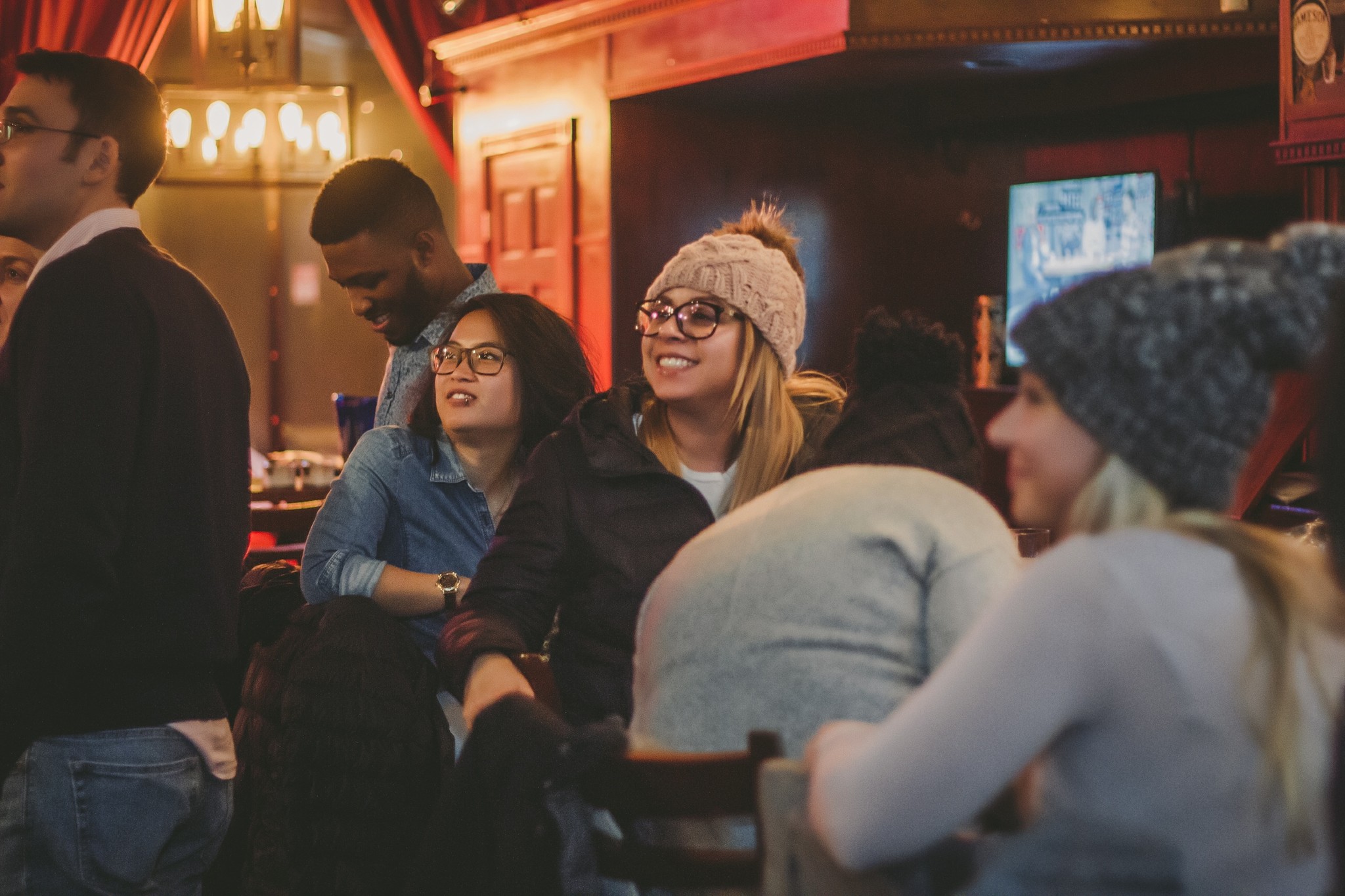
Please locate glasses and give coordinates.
[0,119,102,144]
[426,344,515,376]
[632,299,745,340]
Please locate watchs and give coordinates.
[434,571,460,610]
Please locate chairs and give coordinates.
[759,755,1014,896]
[573,723,782,896]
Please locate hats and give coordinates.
[1009,219,1345,516]
[649,196,807,379]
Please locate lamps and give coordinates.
[205,0,292,87]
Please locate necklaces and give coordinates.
[493,472,515,520]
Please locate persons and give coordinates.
[301,294,595,702]
[800,211,1345,896]
[625,310,1045,865]
[0,235,47,351]
[0,48,252,896]
[304,161,503,433]
[422,189,825,889]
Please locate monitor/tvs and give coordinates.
[1000,171,1161,385]
